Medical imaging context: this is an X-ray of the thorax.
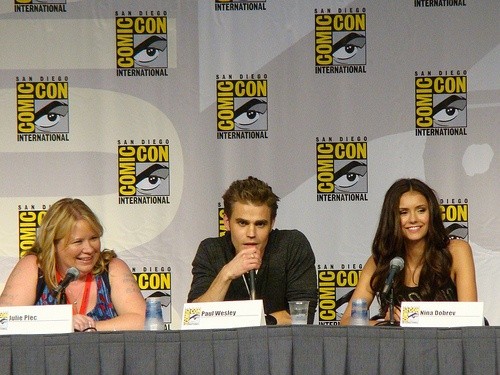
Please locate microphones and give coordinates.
[52,267,80,298]
[248,268,256,300]
[381,257,405,298]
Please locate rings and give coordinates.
[250,253,255,258]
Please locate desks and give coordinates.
[1,324,500,375]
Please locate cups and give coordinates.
[288,301,310,325]
[144,298,165,331]
[348,300,371,325]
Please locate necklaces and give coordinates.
[65,284,84,304]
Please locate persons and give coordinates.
[340,178,489,326]
[0,197,146,332]
[187,176,317,325]
